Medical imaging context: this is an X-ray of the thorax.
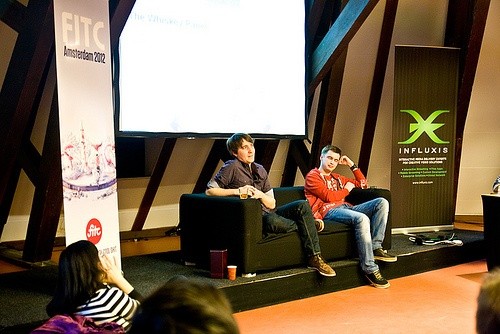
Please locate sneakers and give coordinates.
[364,270,390,288]
[314,219,324,232]
[306,255,336,276]
[373,249,397,262]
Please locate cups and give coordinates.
[360,179,368,189]
[226,265,237,281]
[239,188,248,200]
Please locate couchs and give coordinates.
[179,185,392,278]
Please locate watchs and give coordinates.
[350,164,357,171]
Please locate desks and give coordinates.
[481,194,500,272]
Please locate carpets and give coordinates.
[0,230,482,328]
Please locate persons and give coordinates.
[206,133,336,276]
[304,145,397,289]
[58,240,145,334]
[475,268,500,334]
[124,276,240,334]
